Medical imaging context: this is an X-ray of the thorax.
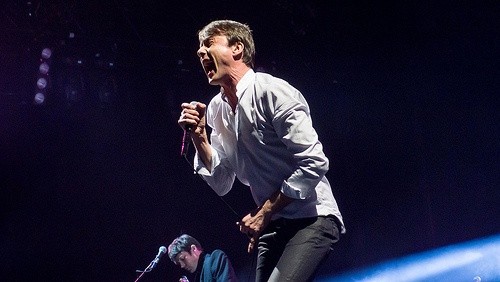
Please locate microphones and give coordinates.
[150,246,167,269]
[181,101,204,155]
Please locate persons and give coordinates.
[168,234,238,281]
[176,18,346,281]
